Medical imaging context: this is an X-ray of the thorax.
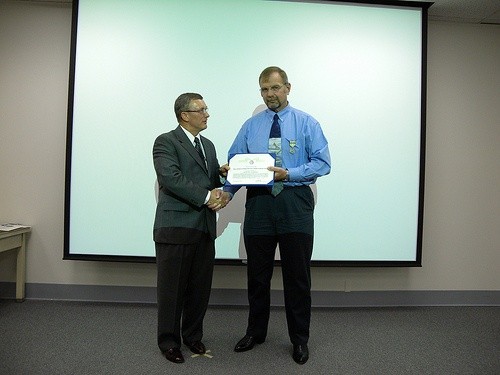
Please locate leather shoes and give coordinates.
[292,344,309,364]
[182,340,206,355]
[234,335,266,352]
[163,348,184,363]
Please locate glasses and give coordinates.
[259,82,288,92]
[181,108,208,113]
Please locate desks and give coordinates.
[0,223,31,303]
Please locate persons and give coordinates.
[152,92,229,365]
[206,66,330,365]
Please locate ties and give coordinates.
[194,137,208,174]
[268,114,284,195]
